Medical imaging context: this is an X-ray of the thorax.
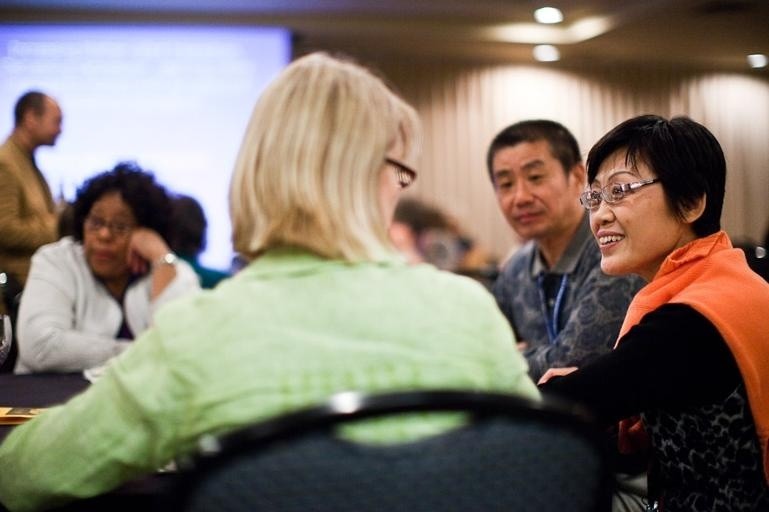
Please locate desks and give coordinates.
[0,368,92,440]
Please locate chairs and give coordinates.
[165,378,621,509]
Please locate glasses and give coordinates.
[578,178,667,210]
[378,150,420,190]
[76,213,137,236]
